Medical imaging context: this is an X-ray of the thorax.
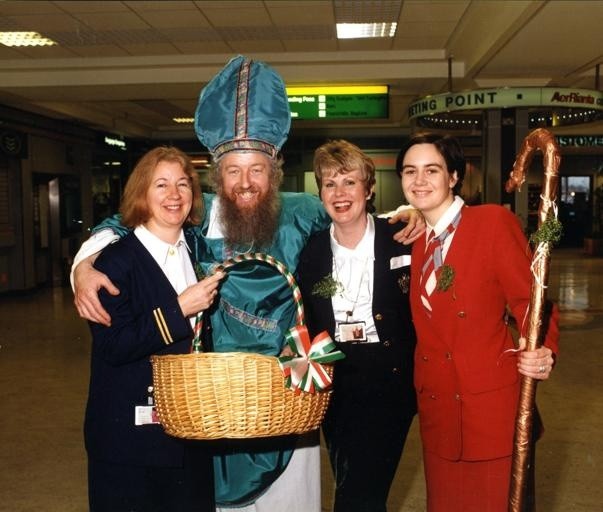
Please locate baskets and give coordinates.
[149,253,334,440]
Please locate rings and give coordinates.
[539,367,546,374]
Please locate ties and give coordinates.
[420,209,461,319]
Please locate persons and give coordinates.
[83,148,229,512]
[409,131,561,511]
[67,52,427,511]
[298,138,418,511]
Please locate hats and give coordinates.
[194,55,291,158]
